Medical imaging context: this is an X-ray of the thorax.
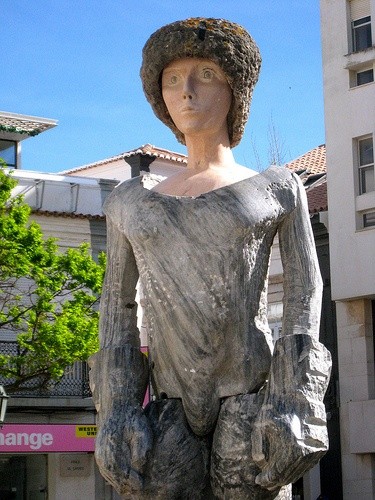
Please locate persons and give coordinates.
[86,17,332,500]
